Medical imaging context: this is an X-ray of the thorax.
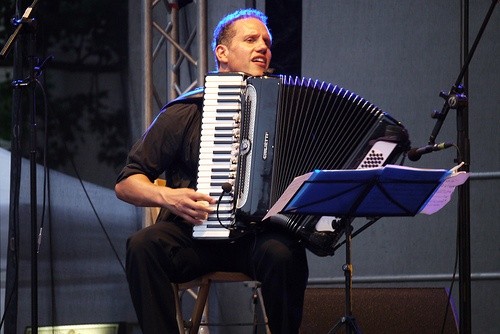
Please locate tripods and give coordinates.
[329,225,360,334]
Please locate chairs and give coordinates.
[150,179,256,334]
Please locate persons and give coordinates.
[111,8,310,334]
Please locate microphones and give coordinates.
[408,143,452,162]
